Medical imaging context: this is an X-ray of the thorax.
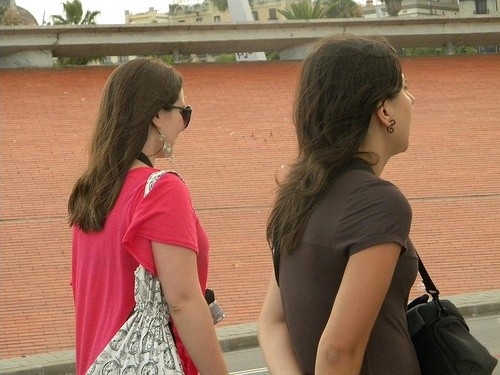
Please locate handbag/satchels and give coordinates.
[84,265,185,375]
[404,293,498,375]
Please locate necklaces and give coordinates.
[136,151,153,168]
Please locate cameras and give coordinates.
[205,289,225,325]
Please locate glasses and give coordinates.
[165,105,192,130]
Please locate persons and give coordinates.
[69,56,230,375]
[255,33,421,375]
